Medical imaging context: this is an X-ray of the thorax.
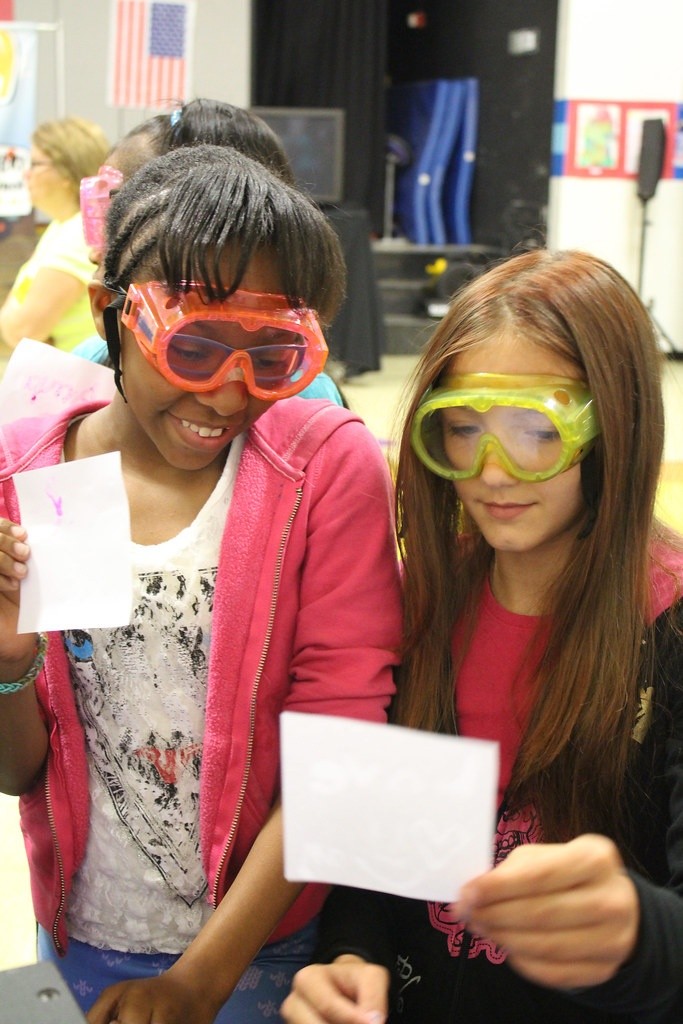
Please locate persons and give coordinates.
[0,101,401,1023]
[280,249,683,1024]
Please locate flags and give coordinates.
[110,0,193,109]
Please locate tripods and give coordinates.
[637,199,679,358]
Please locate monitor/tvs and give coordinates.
[249,107,346,210]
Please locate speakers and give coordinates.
[637,119,667,202]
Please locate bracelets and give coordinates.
[0,632,48,694]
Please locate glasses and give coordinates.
[411,368,604,480]
[121,281,329,412]
[79,167,123,246]
